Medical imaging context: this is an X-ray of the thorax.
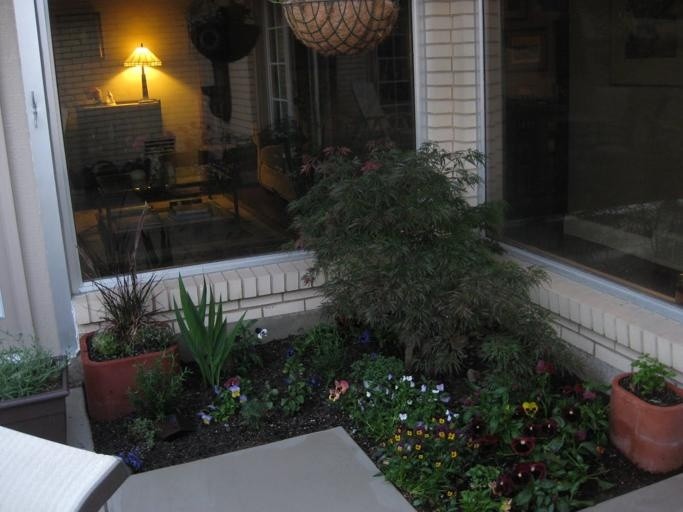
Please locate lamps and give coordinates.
[123,43,162,103]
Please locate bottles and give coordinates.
[104,89,116,106]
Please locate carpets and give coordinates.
[76,189,299,274]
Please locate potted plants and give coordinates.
[80,251,179,421]
[0,326,73,444]
[607,353,683,476]
